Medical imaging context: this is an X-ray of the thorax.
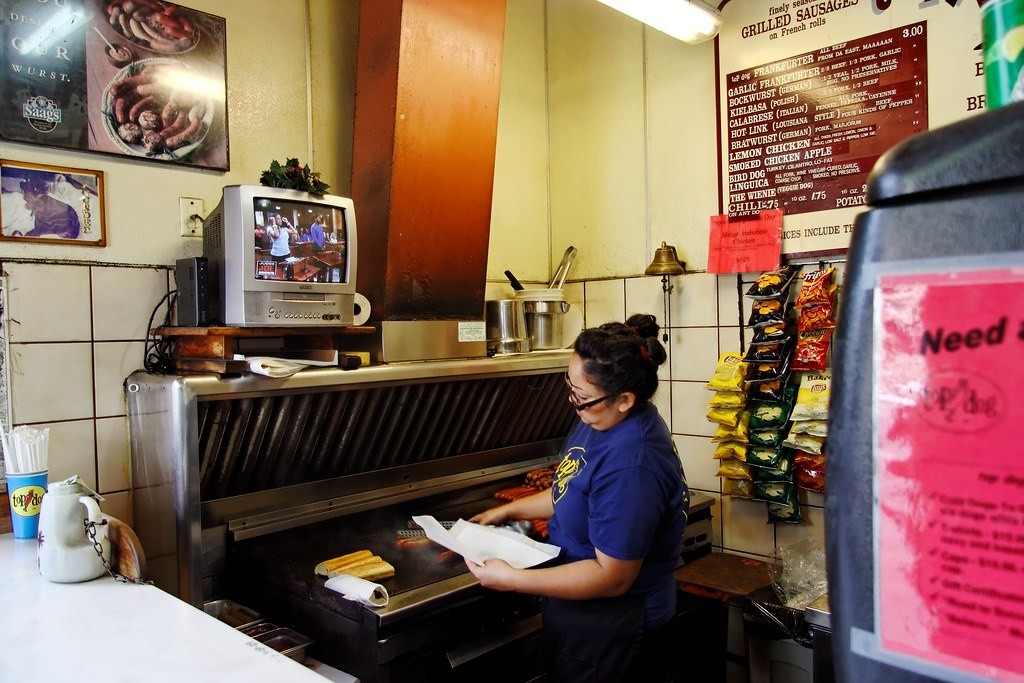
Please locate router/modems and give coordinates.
[176,257,208,327]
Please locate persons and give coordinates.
[464,313,690,683]
[255,212,338,277]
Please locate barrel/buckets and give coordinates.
[484,299,529,354]
[515,288,564,349]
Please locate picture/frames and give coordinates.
[0,159,107,249]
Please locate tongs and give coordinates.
[547,245,578,289]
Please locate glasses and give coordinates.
[564,371,615,411]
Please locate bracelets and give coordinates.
[273,225,277,227]
[286,222,290,225]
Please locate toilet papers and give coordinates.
[324,573,389,607]
[353,292,371,326]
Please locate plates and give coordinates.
[101,57,214,161]
[102,0,201,54]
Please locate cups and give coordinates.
[4,469,48,540]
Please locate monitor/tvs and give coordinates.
[255,260,277,279]
[202,184,357,327]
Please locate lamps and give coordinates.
[588,0,725,47]
